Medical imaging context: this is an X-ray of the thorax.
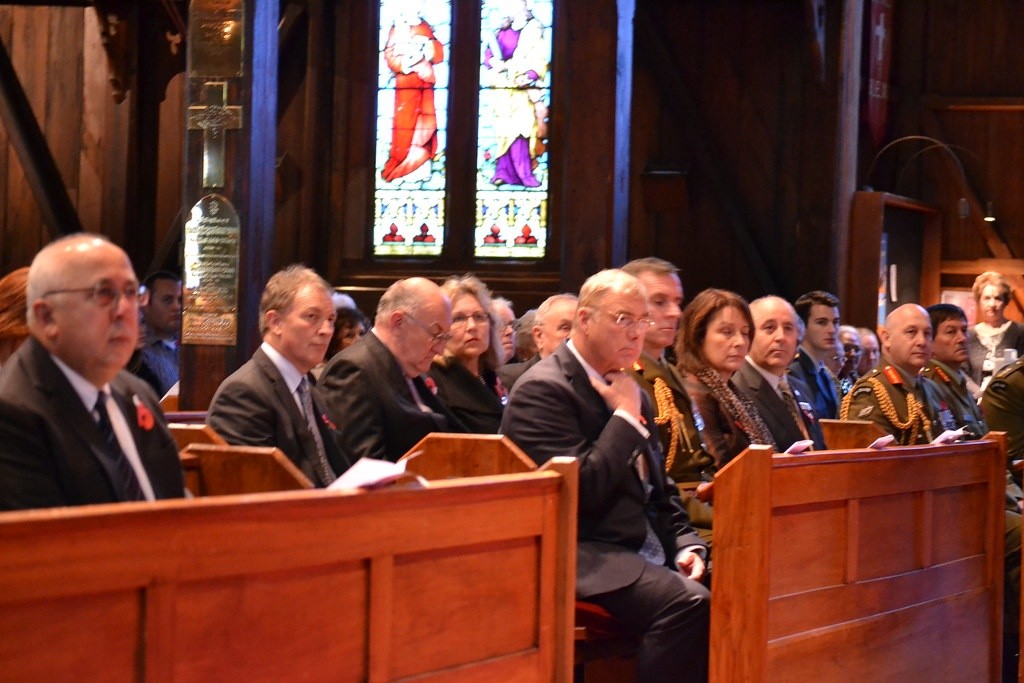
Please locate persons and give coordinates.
[125,272,183,402]
[205,266,356,488]
[0,266,31,367]
[0,234,185,512]
[308,257,1024,624]
[499,269,710,683]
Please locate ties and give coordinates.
[297,377,336,486]
[778,378,814,451]
[638,517,665,565]
[94,390,147,501]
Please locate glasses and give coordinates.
[404,313,454,343]
[826,356,848,362]
[499,320,522,330]
[451,311,490,325]
[589,304,655,332]
[43,278,150,307]
